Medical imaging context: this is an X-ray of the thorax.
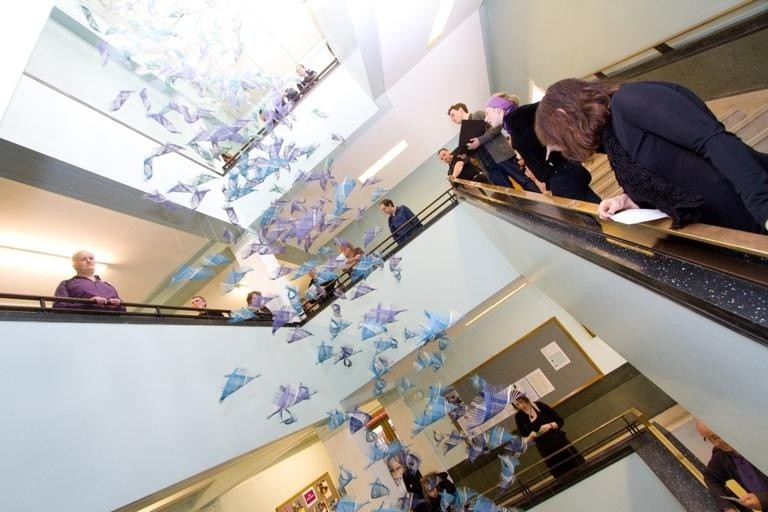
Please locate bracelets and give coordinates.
[549,423,553,429]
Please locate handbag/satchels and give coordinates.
[549,160,592,198]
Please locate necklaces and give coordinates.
[523,406,533,418]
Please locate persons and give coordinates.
[245,291,272,320]
[438,92,603,204]
[403,462,460,512]
[304,270,336,306]
[259,64,318,122]
[191,296,224,317]
[341,242,365,277]
[696,418,768,512]
[380,200,424,247]
[221,152,240,167]
[509,390,586,479]
[534,79,768,236]
[53,251,126,311]
